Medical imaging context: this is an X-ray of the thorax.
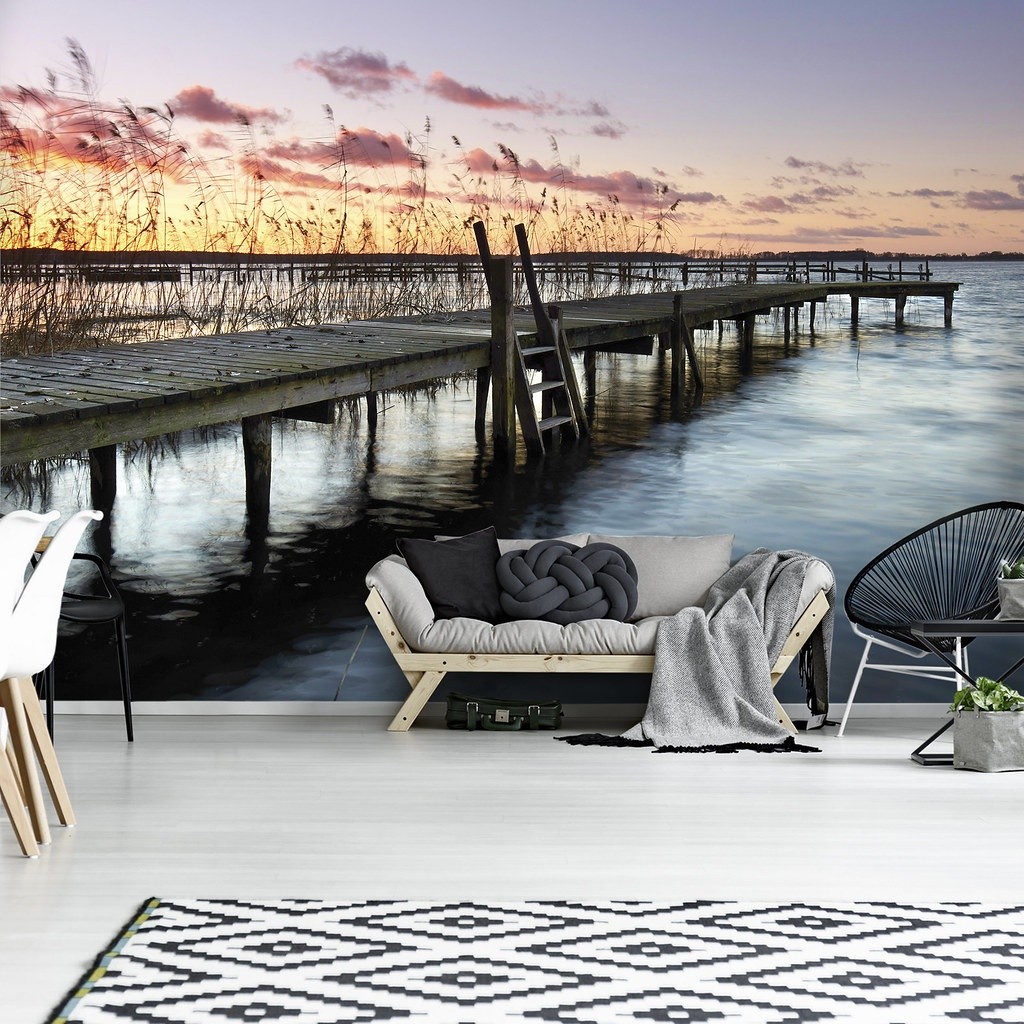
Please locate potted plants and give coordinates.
[947,676,1024,773]
[993,555,1024,621]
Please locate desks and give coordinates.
[910,620,1024,765]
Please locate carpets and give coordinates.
[43,895,1024,1024]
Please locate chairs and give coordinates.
[834,501,1024,738]
[0,509,133,859]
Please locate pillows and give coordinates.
[433,532,587,557]
[396,526,501,618]
[587,534,734,624]
[496,540,639,625]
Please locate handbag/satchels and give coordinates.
[446,691,564,732]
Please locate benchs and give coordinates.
[364,525,831,735]
